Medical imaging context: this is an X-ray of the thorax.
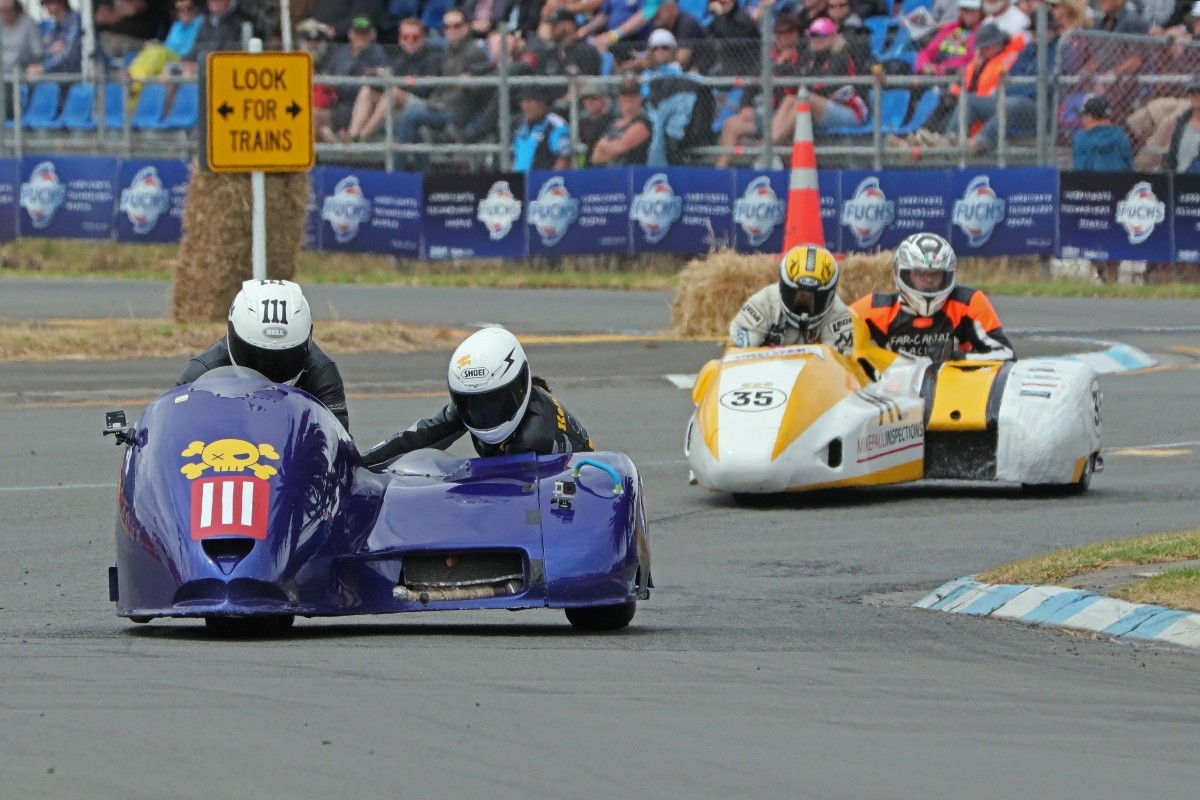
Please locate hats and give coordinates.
[582,82,607,96]
[546,9,575,22]
[958,0,980,10]
[653,5,679,25]
[809,18,837,34]
[772,12,800,32]
[648,29,678,49]
[517,86,545,98]
[971,24,1008,48]
[348,15,373,33]
[1185,71,1200,94]
[619,80,639,92]
[1078,94,1113,117]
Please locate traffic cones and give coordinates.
[778,89,827,256]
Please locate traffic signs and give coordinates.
[205,50,316,173]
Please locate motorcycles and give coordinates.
[683,342,1105,503]
[102,364,655,640]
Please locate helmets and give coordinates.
[779,244,840,332]
[446,328,531,444]
[892,232,958,318]
[225,279,313,387]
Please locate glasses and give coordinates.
[443,23,464,30]
[400,34,422,41]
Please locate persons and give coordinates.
[0,0,1200,174]
[171,280,351,436]
[353,324,595,476]
[848,231,1017,367]
[728,242,856,360]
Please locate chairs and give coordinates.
[0,0,1200,147]
